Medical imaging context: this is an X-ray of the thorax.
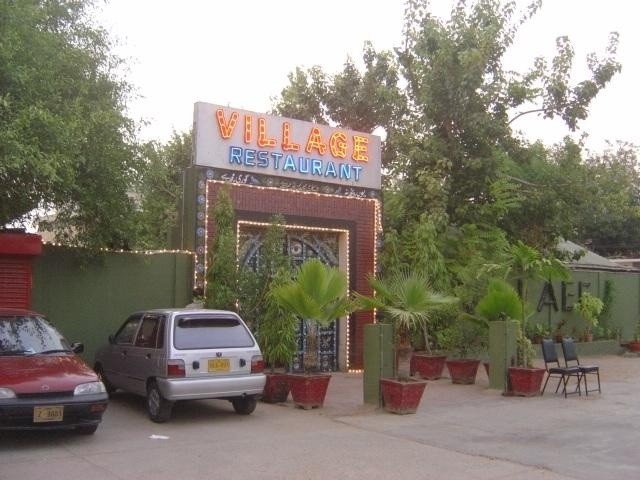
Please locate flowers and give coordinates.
[555,317,569,334]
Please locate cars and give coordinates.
[93,307,269,424]
[1,307,110,436]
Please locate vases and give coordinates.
[261,268,296,407]
[408,287,450,382]
[350,271,454,420]
[474,277,533,387]
[555,333,563,342]
[506,301,544,398]
[268,258,346,411]
[438,311,486,387]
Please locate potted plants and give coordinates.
[628,333,640,353]
[571,292,605,340]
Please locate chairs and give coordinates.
[541,337,602,397]
